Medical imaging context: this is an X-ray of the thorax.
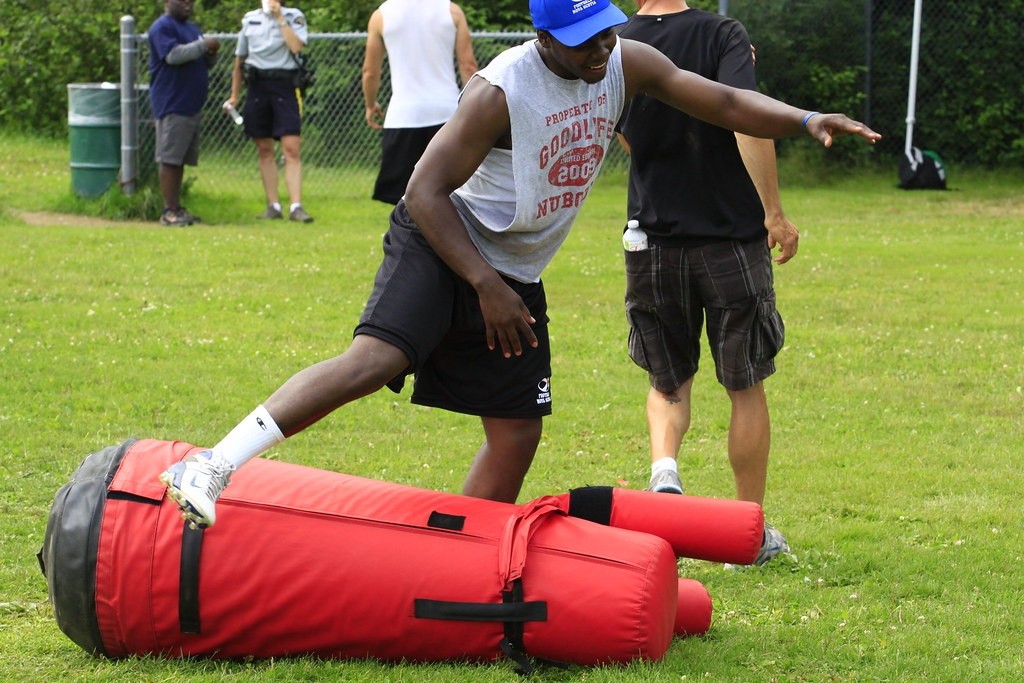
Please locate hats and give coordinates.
[529,1,629,49]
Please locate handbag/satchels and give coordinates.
[897,152,948,188]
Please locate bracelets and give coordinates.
[278,20,289,27]
[800,112,819,127]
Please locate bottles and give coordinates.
[621,219,648,253]
[223,101,244,126]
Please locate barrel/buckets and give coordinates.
[66,76,160,203]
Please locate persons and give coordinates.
[221,0,317,225]
[358,0,481,209]
[161,0,882,530]
[607,0,800,573]
[141,1,226,231]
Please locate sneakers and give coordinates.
[644,456,687,498]
[160,445,232,533]
[729,521,784,569]
[288,204,315,223]
[257,206,282,222]
[158,204,200,226]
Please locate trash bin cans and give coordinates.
[67,82,158,200]
